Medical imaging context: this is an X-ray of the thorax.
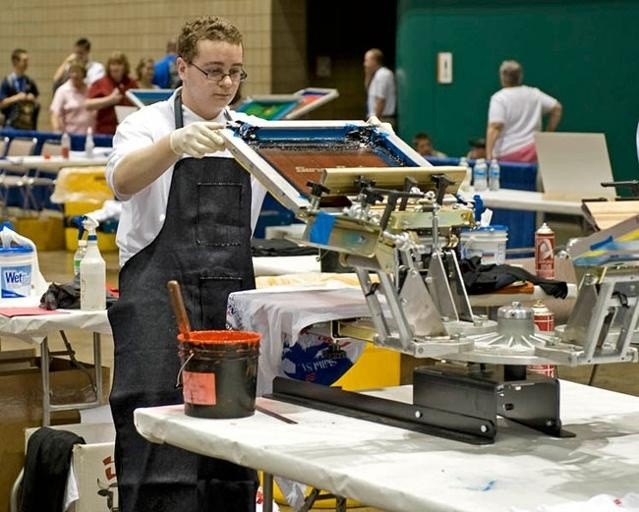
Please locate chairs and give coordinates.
[1,132,113,217]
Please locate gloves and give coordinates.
[170,121,225,160]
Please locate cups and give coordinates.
[6,155,24,165]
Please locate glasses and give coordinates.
[190,62,248,82]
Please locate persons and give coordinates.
[410,131,449,163]
[464,137,486,162]
[362,47,400,135]
[0,36,180,135]
[102,14,400,512]
[484,59,564,168]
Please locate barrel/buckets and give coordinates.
[460,225,509,266]
[1,225,39,299]
[180,329,262,418]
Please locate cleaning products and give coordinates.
[68,211,107,312]
[85,126,95,157]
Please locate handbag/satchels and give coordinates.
[8,100,40,130]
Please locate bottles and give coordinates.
[497,300,558,382]
[73,236,107,311]
[458,156,499,192]
[42,128,96,159]
